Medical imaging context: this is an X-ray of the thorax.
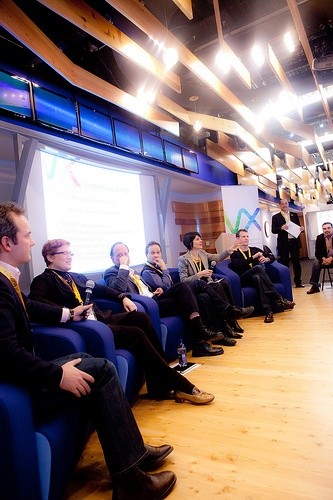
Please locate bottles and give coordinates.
[178,338,187,367]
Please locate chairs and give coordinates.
[322,264,333,290]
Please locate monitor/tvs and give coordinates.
[163,139,184,169]
[139,128,165,163]
[181,147,199,174]
[76,100,115,147]
[0,69,33,122]
[32,81,80,136]
[112,117,143,155]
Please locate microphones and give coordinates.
[82,280,95,315]
[209,261,216,270]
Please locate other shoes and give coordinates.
[307,285,320,294]
[274,298,296,309]
[192,340,224,358]
[229,320,244,333]
[295,284,305,288]
[147,390,174,401]
[229,306,255,319]
[212,336,237,346]
[264,312,274,323]
[193,328,224,343]
[221,325,243,339]
[174,386,215,404]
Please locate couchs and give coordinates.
[1,261,293,500]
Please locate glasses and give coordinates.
[51,251,73,257]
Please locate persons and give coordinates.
[272,199,305,288]
[27,239,214,405]
[230,229,296,323]
[139,241,254,346]
[306,222,333,295]
[0,201,178,500]
[179,232,245,339]
[102,242,224,356]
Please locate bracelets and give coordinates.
[69,308,74,321]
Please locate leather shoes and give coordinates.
[111,468,176,500]
[130,442,173,472]
